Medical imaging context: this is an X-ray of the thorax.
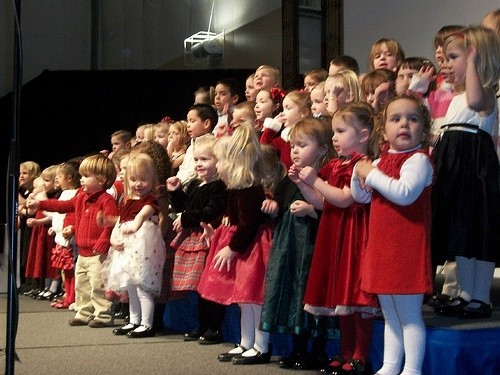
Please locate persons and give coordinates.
[16,11,500,375]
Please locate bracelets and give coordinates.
[328,98,337,101]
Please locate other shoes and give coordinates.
[69,318,84,325]
[23,289,40,296]
[51,292,70,308]
[89,320,107,327]
[434,294,491,319]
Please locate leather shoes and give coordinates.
[125,316,130,321]
[199,329,224,343]
[114,312,127,319]
[38,290,56,300]
[320,356,347,374]
[33,290,48,299]
[232,347,272,364]
[184,328,208,341]
[332,359,368,375]
[218,345,247,361]
[112,323,140,334]
[294,355,327,369]
[279,353,302,368]
[126,324,156,338]
